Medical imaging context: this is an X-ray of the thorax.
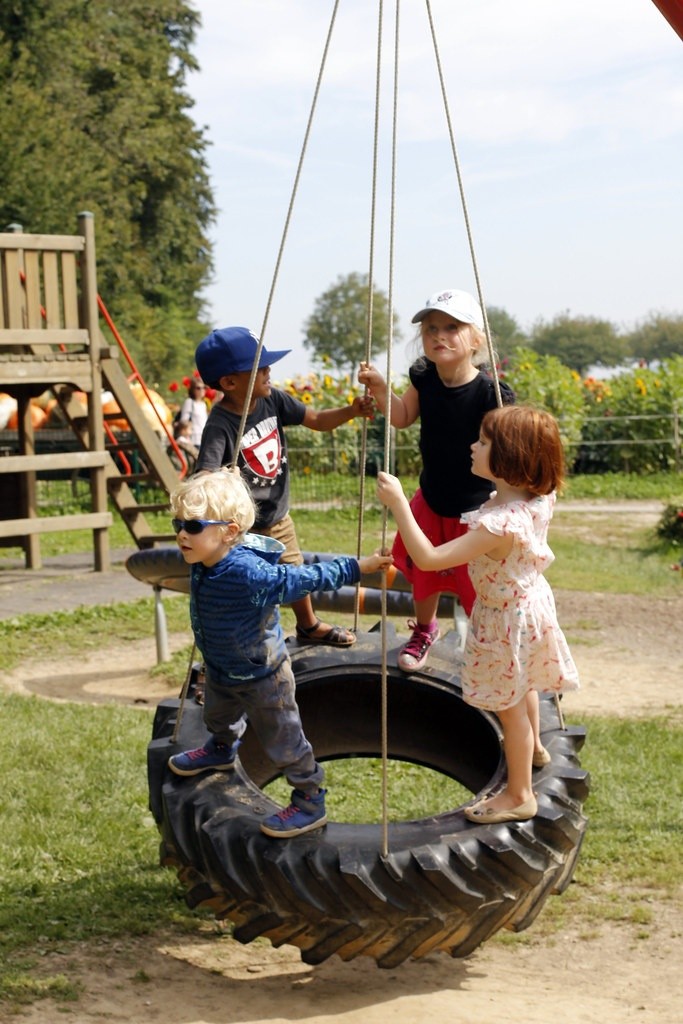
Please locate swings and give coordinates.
[147,0,592,971]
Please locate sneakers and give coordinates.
[260,788,327,837]
[168,738,240,776]
[397,619,440,672]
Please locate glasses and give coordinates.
[194,387,206,390]
[171,519,232,534]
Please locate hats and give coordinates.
[410,290,484,332]
[194,326,292,383]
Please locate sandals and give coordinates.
[194,675,205,705]
[296,619,357,645]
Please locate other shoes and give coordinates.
[501,737,551,766]
[464,799,537,824]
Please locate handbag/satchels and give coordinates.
[176,420,192,437]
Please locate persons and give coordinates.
[169,470,394,838]
[357,291,516,673]
[196,326,375,647]
[171,379,209,470]
[378,405,580,823]
